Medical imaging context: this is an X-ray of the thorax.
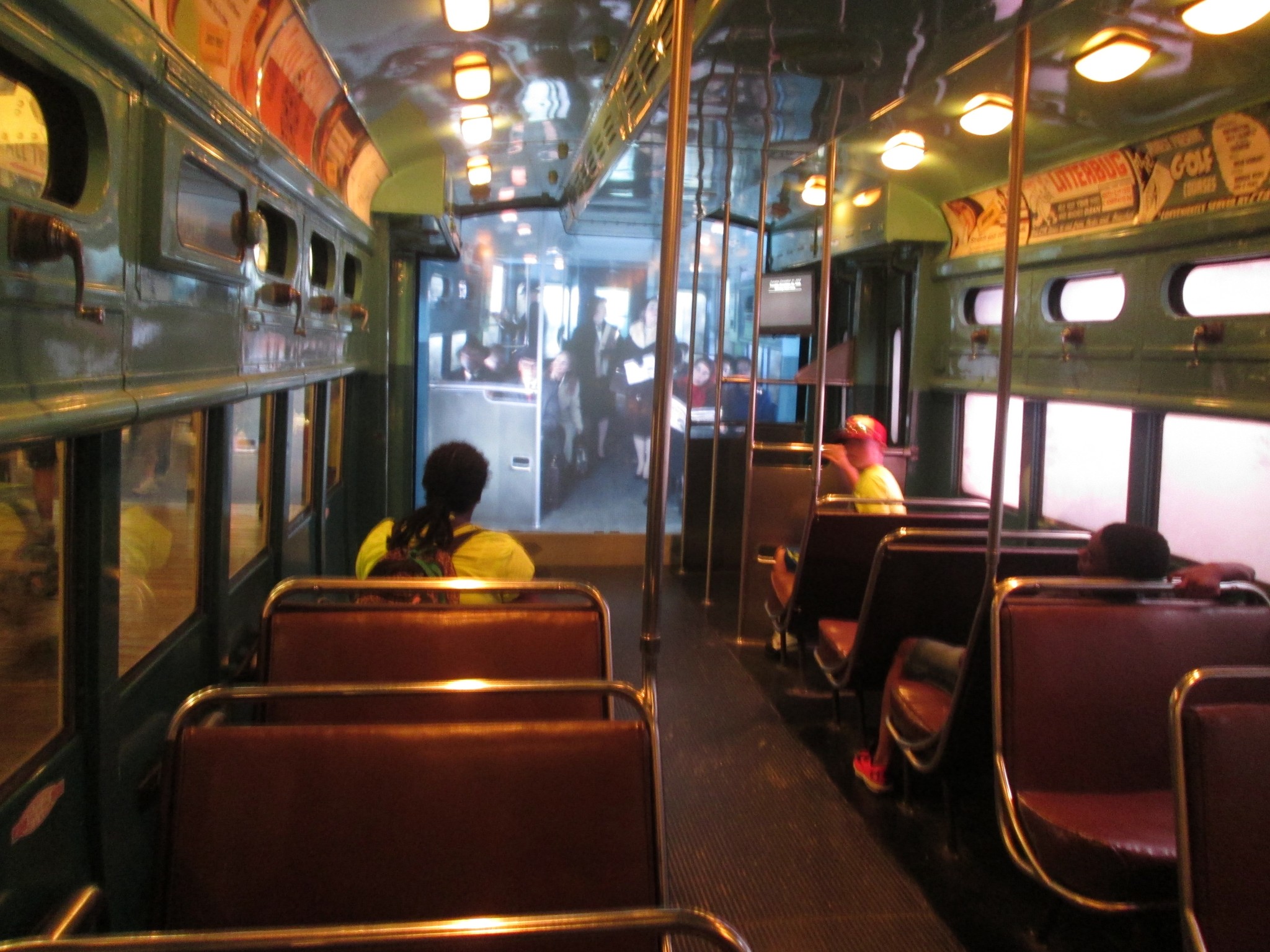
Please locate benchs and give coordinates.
[813,525,1094,736]
[886,577,1269,951]
[251,578,617,723]
[155,676,678,951]
[766,494,990,693]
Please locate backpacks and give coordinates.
[358,517,459,607]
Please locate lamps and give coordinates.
[958,99,1014,136]
[453,63,492,101]
[1179,0,1270,36]
[1073,33,1152,83]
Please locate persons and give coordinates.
[449,280,771,484]
[772,414,907,650]
[0,409,200,595]
[850,522,1256,793]
[357,439,545,604]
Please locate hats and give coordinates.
[838,415,888,446]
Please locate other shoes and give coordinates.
[765,643,794,664]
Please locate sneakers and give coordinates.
[853,749,889,792]
